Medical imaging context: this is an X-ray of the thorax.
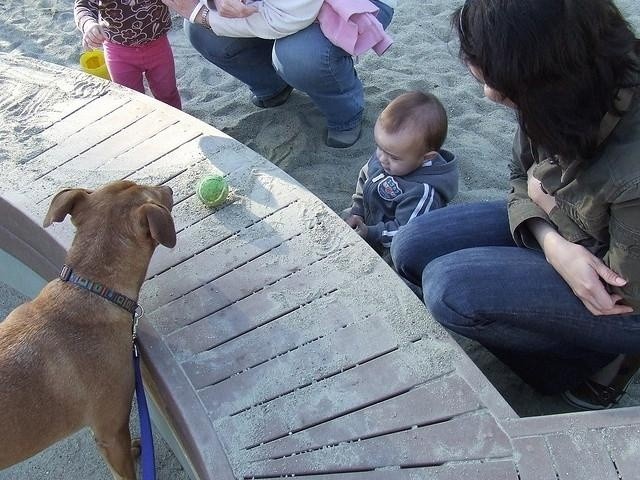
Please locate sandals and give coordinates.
[561,350,640,410]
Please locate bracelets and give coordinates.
[189,2,204,24]
[202,7,212,31]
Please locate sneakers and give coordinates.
[327,124,361,148]
[252,86,293,107]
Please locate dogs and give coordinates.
[0,178,178,480]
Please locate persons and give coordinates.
[161,0,395,148]
[73,0,181,112]
[337,91,459,270]
[390,0,640,411]
